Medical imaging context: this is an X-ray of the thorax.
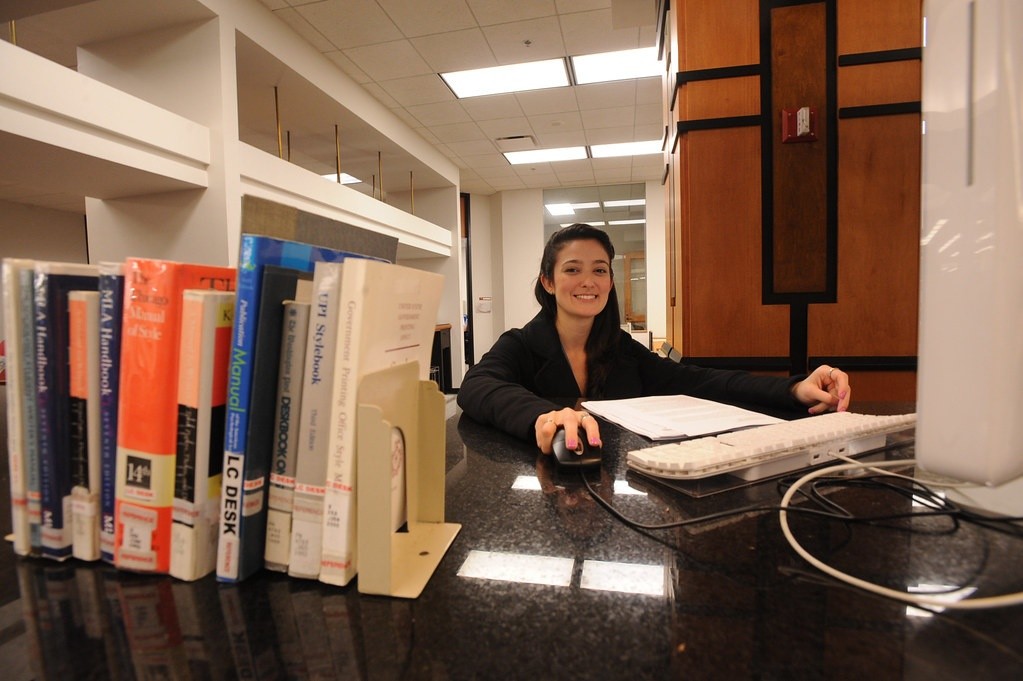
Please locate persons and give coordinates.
[458,409,620,549]
[456,223,851,456]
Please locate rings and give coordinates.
[829,368,835,378]
[581,415,592,426]
[545,420,558,427]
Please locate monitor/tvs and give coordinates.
[911,0,1023,519]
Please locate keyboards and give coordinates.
[626,412,917,483]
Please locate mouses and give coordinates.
[550,427,601,467]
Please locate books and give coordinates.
[0,233,446,587]
